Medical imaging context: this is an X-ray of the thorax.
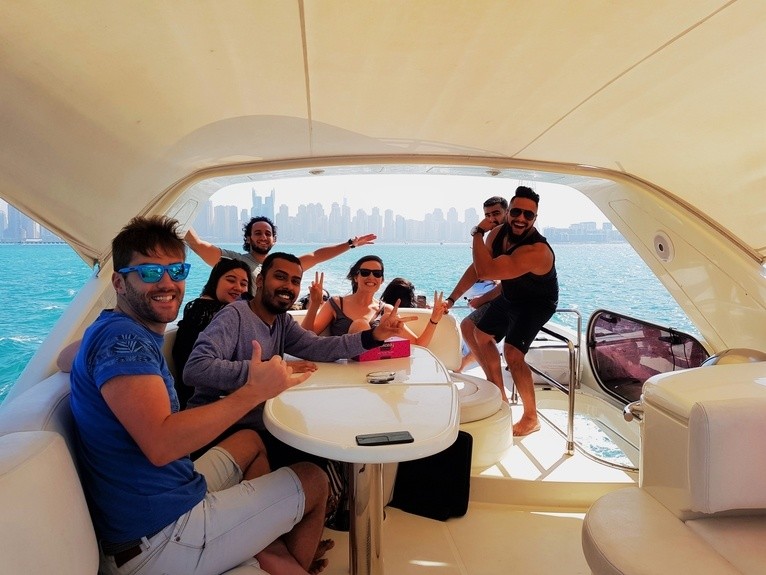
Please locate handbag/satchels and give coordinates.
[390,431,474,522]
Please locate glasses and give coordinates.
[357,268,384,278]
[509,207,536,221]
[119,263,191,283]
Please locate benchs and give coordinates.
[0,303,511,575]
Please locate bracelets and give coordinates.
[430,318,438,325]
[446,297,455,305]
[348,239,356,248]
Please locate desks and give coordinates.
[263,340,459,575]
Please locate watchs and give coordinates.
[470,226,485,238]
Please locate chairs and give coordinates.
[582,364,766,575]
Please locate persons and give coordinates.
[183,252,419,575]
[171,216,448,410]
[67,216,328,575]
[442,186,560,435]
